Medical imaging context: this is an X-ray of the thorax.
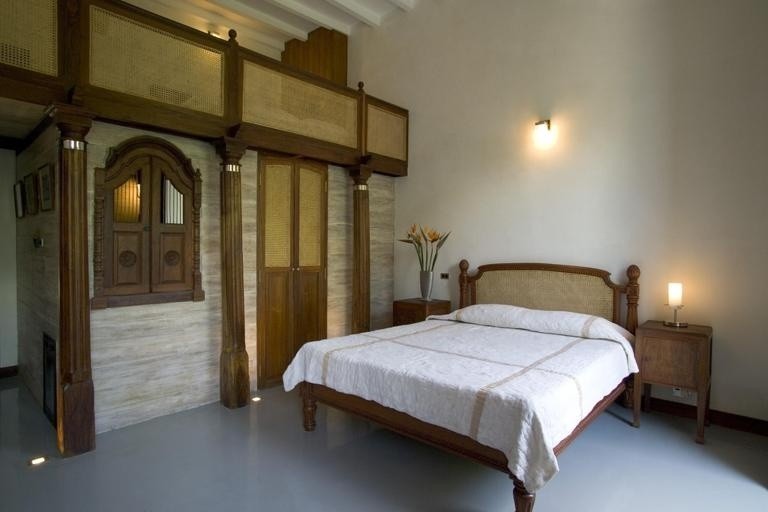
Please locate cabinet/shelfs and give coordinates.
[633,319,713,444]
[392,298,451,326]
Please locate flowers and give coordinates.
[397,222,452,271]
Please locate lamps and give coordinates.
[663,282,688,327]
[533,119,557,149]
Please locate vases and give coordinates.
[420,271,434,302]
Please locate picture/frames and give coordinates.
[36,162,55,212]
[23,173,37,216]
[13,180,24,219]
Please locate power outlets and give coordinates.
[672,387,682,397]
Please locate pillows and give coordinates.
[456,302,610,341]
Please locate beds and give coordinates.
[282,259,641,512]
